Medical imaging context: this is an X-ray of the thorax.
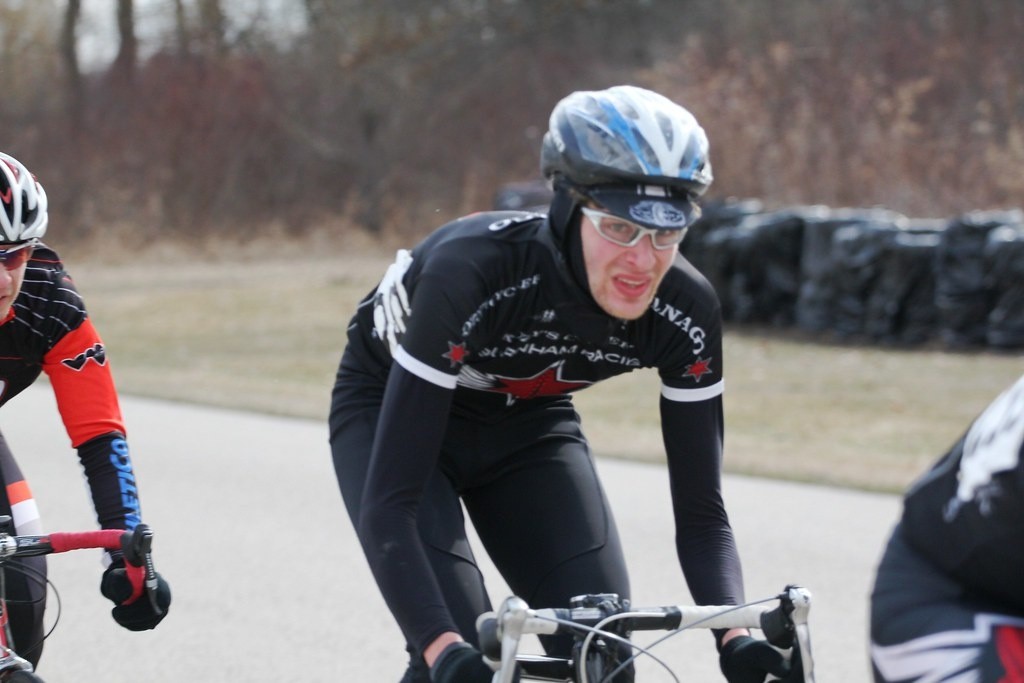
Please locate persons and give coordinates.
[329,86,784,683]
[870,375,1024,683]
[0,152,171,683]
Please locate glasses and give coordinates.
[0,238,37,270]
[582,206,688,250]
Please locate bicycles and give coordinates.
[471,588,819,683]
[1,511,168,683]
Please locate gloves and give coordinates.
[720,636,789,683]
[431,642,495,683]
[101,551,171,631]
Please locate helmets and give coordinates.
[541,85,713,227]
[0,151,48,246]
